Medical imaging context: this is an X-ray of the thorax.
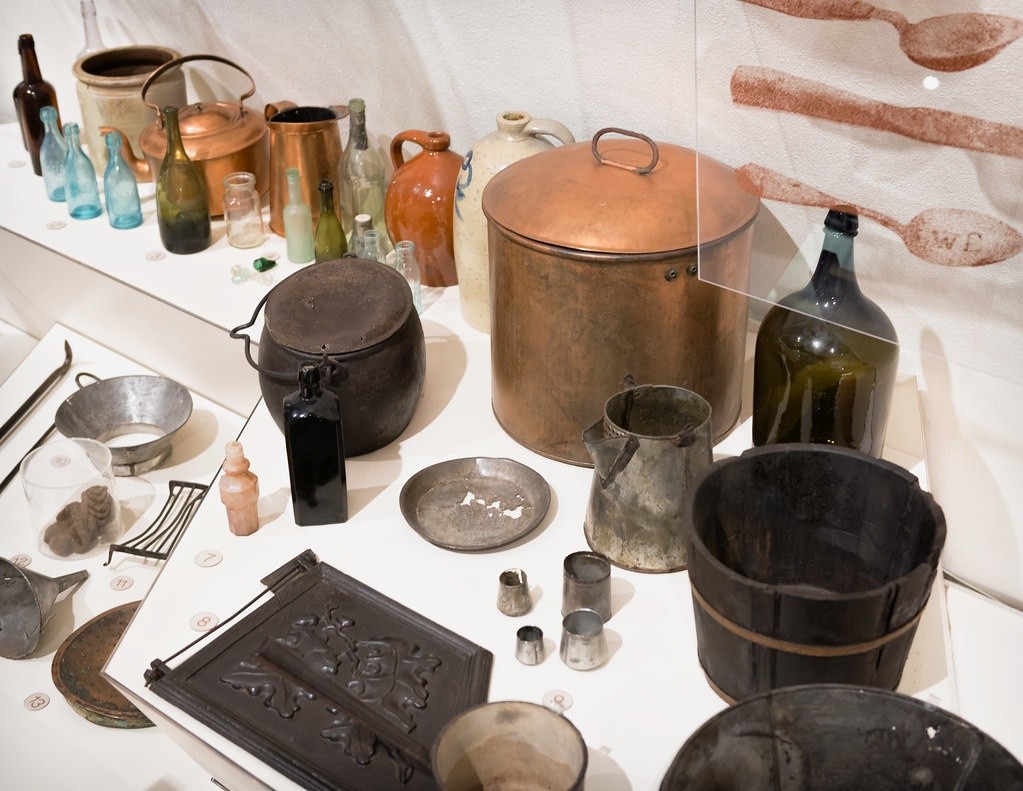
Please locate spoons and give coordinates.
[735,163,1023,266]
[737,0,1023,72]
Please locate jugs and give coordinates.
[581,384,713,576]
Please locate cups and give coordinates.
[499,551,611,671]
[20,436,122,561]
[430,701,589,791]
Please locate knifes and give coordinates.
[730,65,1023,162]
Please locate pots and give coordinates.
[482,128,760,472]
[229,250,425,458]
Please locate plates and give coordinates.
[400,458,552,553]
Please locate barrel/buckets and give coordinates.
[686,442,946,708]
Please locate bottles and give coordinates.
[219,360,348,537]
[754,201,899,456]
[13,34,577,323]
